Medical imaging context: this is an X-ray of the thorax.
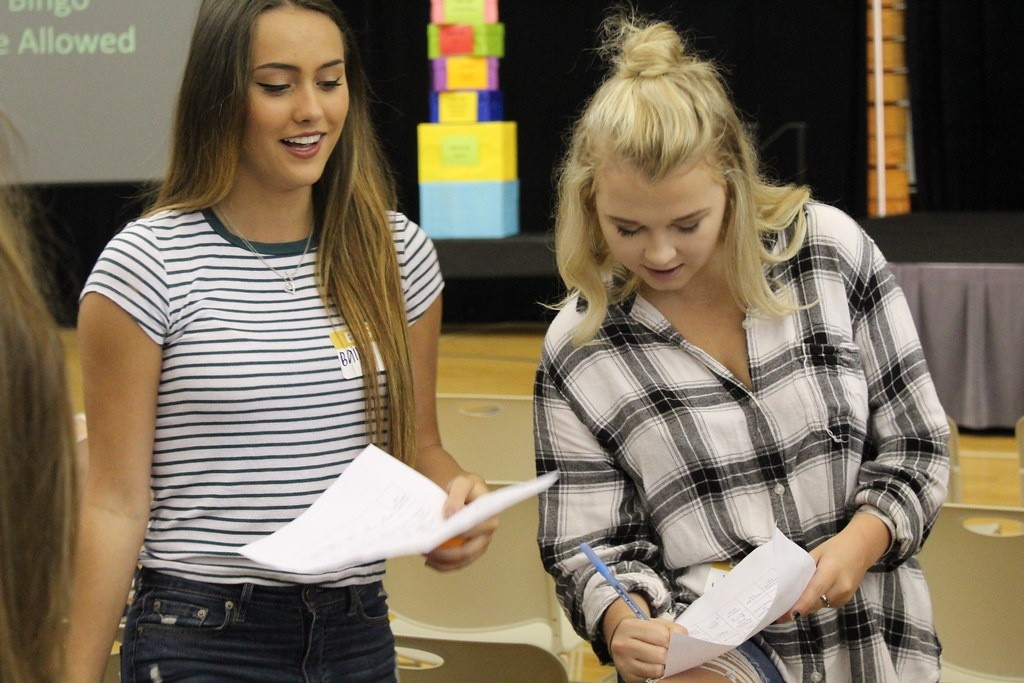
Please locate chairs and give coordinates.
[383,395,618,683]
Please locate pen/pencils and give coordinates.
[581,541,649,620]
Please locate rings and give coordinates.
[820,594,831,609]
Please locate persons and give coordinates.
[526,18,954,682]
[56,0,496,683]
